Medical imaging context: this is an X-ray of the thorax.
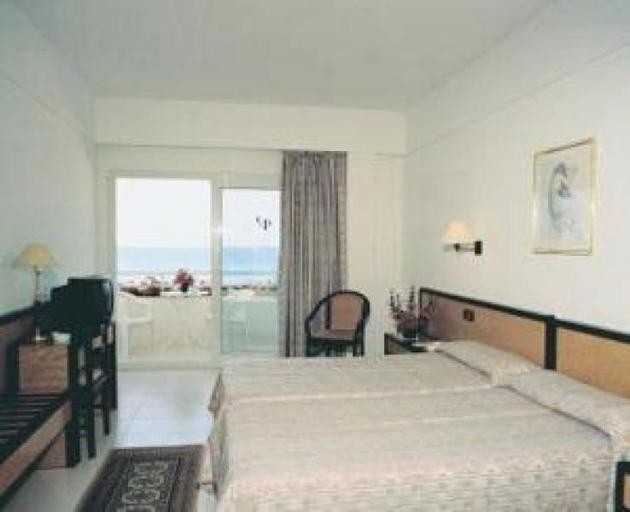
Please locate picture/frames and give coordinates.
[528,135,599,257]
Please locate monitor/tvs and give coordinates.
[50,275,114,338]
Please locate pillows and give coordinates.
[510,368,630,435]
[446,340,540,389]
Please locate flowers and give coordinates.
[392,288,437,332]
[173,269,194,287]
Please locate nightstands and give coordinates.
[383,332,438,355]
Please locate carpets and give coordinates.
[73,443,203,512]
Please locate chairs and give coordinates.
[33,276,116,457]
[305,290,372,357]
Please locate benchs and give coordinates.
[1,338,82,494]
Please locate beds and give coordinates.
[207,286,555,423]
[210,320,630,512]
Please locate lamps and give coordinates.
[16,242,55,302]
[441,221,485,256]
